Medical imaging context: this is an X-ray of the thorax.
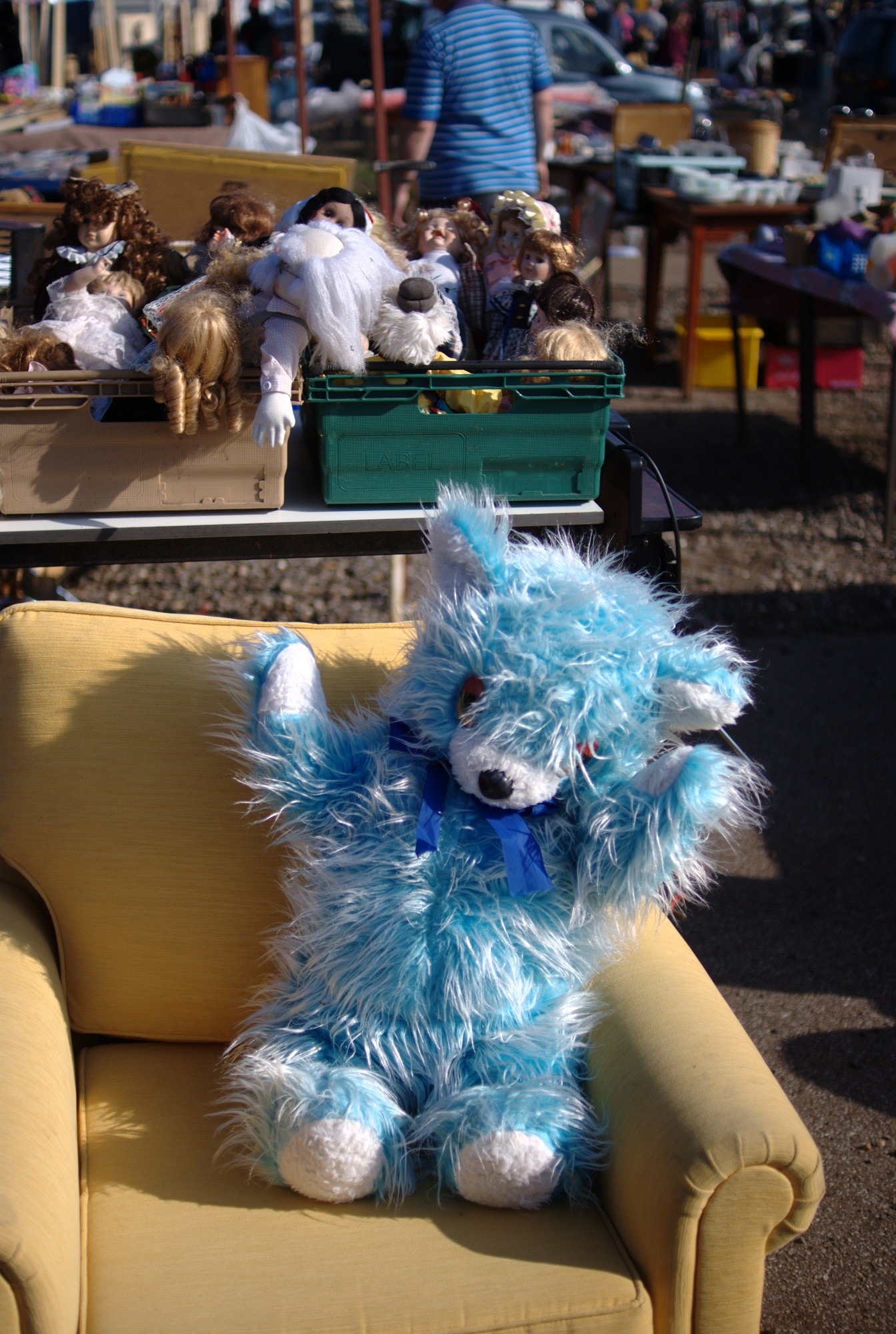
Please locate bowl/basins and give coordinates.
[670,164,804,206]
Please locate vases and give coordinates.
[723,121,783,178]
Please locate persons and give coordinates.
[196,2,368,116]
[593,0,878,116]
[389,0,559,250]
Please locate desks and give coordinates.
[0,484,608,598]
[547,145,896,546]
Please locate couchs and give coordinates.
[2,597,827,1334]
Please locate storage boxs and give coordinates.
[2,354,304,522]
[304,340,627,509]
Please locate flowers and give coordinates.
[719,86,794,122]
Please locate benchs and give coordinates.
[66,135,361,233]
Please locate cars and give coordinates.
[517,10,714,140]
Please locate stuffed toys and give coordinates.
[214,488,765,1207]
[1,173,608,444]
